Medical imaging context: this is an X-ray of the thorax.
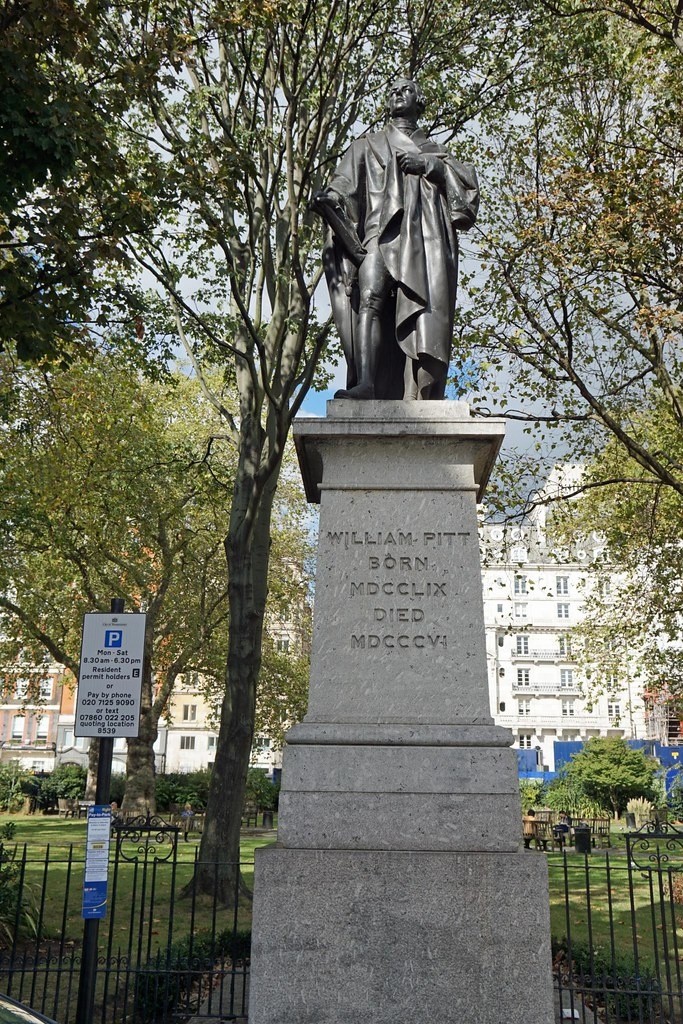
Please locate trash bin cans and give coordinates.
[263,810,273,829]
[574,824,591,854]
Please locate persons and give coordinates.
[553,810,573,846]
[308,77,482,401]
[110,801,119,823]
[523,808,540,851]
[180,804,194,844]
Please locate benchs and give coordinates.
[172,813,206,837]
[168,803,205,822]
[241,801,259,827]
[111,804,143,836]
[568,818,611,848]
[528,809,558,833]
[522,819,563,852]
[57,799,87,818]
